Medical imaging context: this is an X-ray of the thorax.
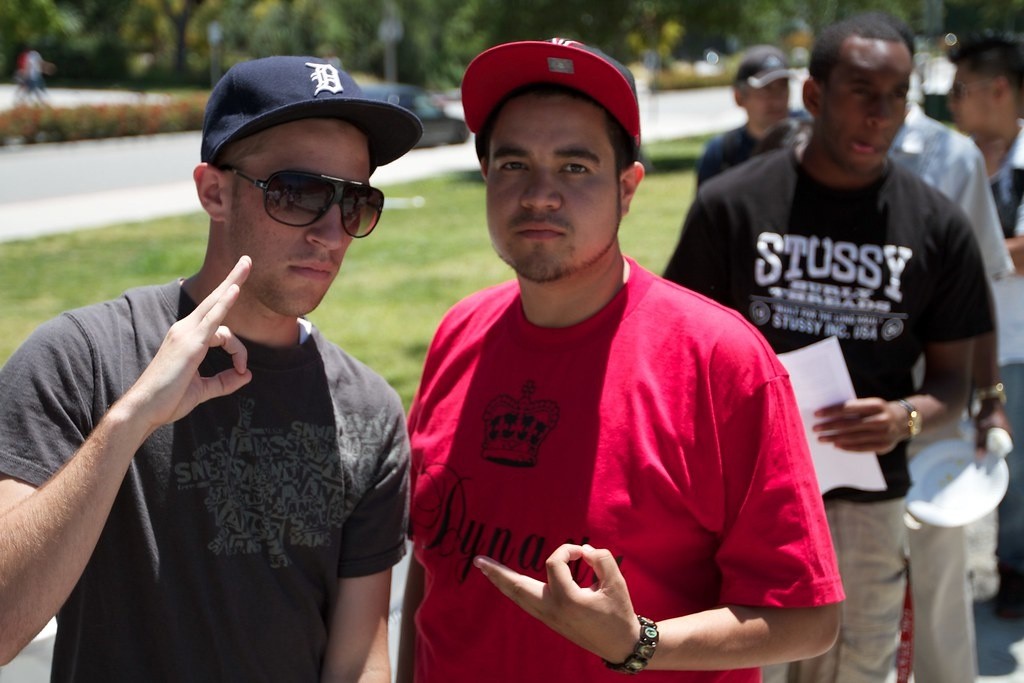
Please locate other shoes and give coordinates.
[994,563,1024,618]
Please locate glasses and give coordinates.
[949,78,996,98]
[218,164,385,238]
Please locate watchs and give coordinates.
[896,399,921,439]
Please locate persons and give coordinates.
[888,20,1015,683]
[14,48,54,109]
[947,34,1023,622]
[0,56,408,683]
[397,37,846,683]
[696,47,813,195]
[662,17,992,683]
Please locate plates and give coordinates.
[905,437,1011,528]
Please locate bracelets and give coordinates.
[601,615,659,675]
[982,383,1007,405]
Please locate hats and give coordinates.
[461,38,641,161]
[735,45,797,89]
[201,56,423,176]
